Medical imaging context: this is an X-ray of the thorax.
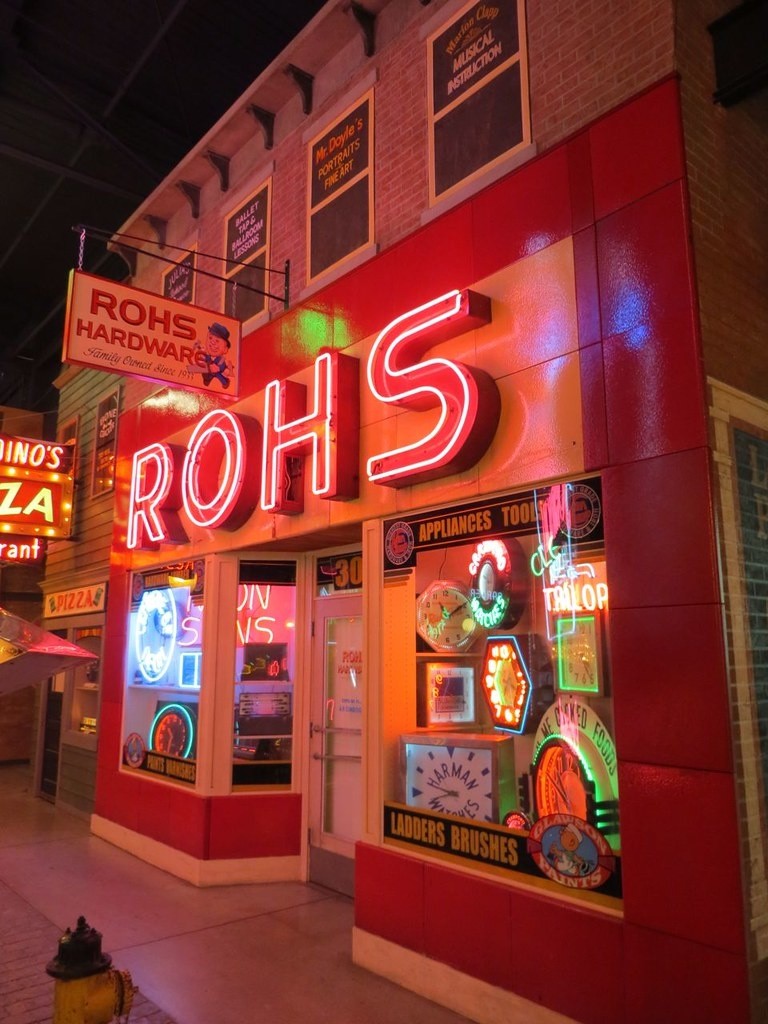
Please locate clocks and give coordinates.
[416,579,486,653]
[478,634,555,735]
[552,609,607,699]
[398,732,517,825]
[419,661,479,727]
[467,538,530,630]
[148,703,197,759]
[518,697,621,850]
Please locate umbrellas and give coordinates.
[0,607,99,694]
